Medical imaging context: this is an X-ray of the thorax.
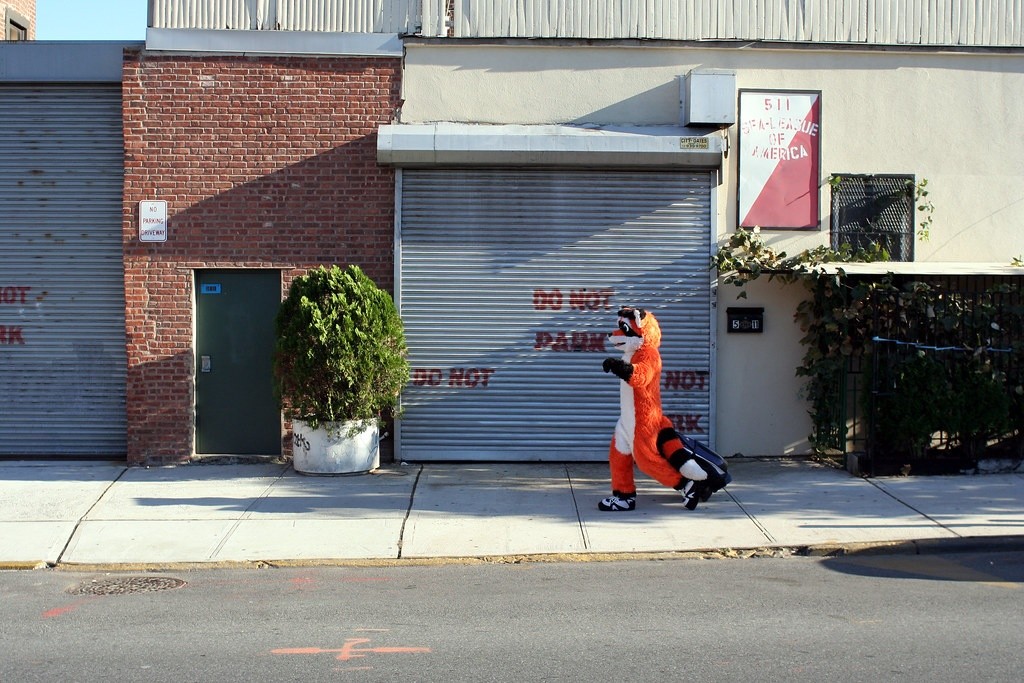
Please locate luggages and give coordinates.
[676,431,732,501]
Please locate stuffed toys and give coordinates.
[601,307,713,512]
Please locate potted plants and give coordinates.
[272,263,410,476]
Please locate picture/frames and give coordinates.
[737,87,822,231]
